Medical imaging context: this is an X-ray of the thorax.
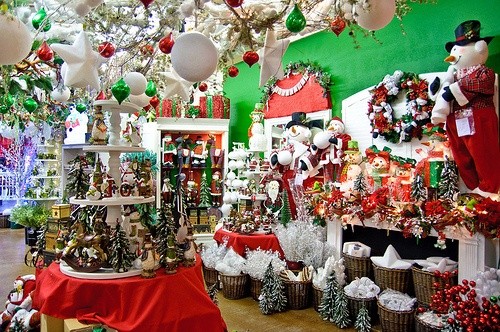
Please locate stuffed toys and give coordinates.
[129,207,143,235]
[366,149,390,190]
[428,21,500,201]
[248,103,267,150]
[123,172,134,185]
[391,163,412,185]
[311,117,352,184]
[0,276,40,332]
[269,111,318,190]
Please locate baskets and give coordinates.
[201,252,459,332]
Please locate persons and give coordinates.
[340,140,364,193]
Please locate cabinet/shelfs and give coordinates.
[22,100,268,279]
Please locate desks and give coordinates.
[30,252,227,332]
[214,225,285,259]
[325,216,500,285]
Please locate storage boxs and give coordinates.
[52,204,70,218]
[47,217,72,233]
[46,233,69,250]
